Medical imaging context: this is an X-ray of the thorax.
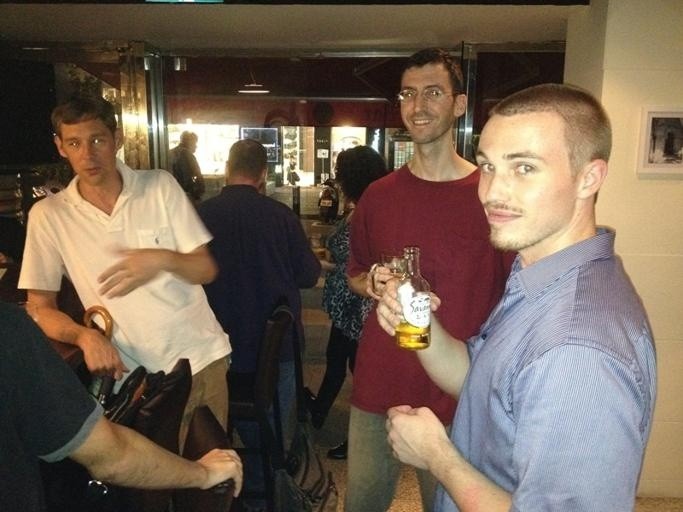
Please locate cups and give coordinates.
[381,256,407,280]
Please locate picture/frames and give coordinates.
[636,107,683,174]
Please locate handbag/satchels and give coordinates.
[272,423,338,512]
[41,365,167,512]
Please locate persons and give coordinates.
[295,144,389,460]
[0,262,245,512]
[375,79,660,511]
[334,43,520,510]
[10,95,233,447]
[192,136,323,481]
[164,130,206,203]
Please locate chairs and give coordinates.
[226,295,290,512]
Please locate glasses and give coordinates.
[398,87,460,102]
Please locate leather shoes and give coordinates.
[303,385,325,428]
[328,437,348,461]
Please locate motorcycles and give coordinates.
[319,176,338,222]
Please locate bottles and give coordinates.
[396,246,431,351]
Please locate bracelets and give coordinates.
[363,261,382,301]
